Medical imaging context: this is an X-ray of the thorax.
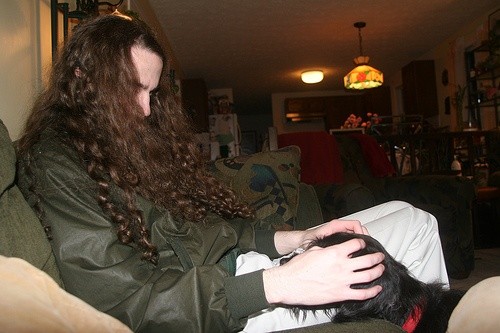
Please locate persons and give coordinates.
[0,9,500,333]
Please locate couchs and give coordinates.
[0,120,474,333]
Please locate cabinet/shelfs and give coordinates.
[401,59,438,118]
[285,86,392,134]
[464,35,500,129]
[181,78,209,134]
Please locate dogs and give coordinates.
[280,231,464,333]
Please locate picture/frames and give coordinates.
[488,8,500,56]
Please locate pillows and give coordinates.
[204,144,301,231]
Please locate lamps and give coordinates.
[344,21,383,90]
